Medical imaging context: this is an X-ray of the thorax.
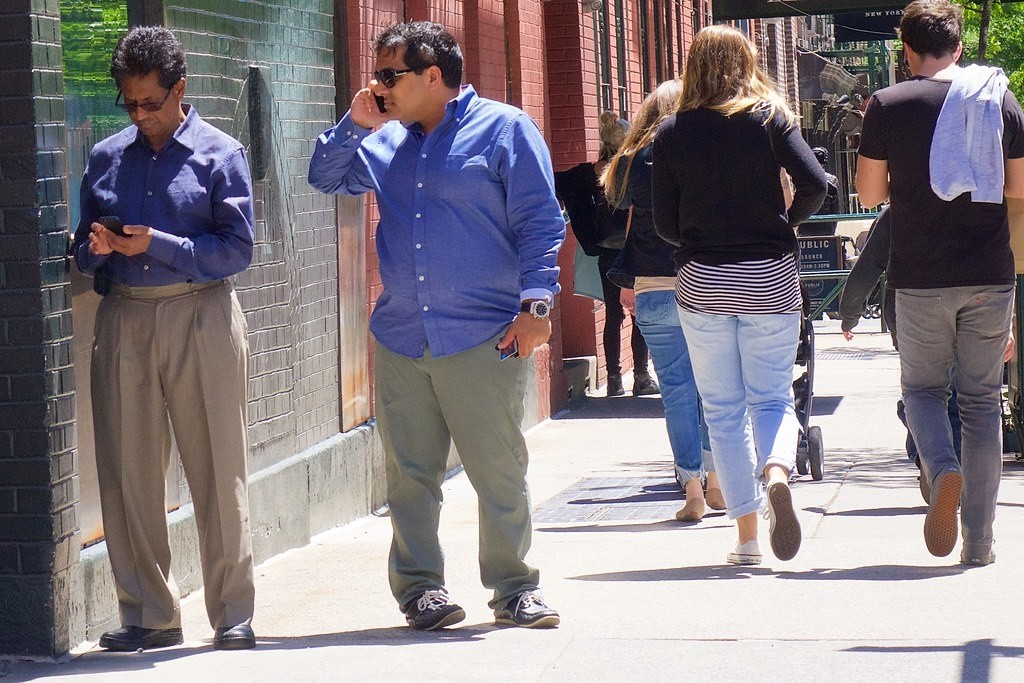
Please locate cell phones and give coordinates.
[98,216,133,239]
[371,91,388,116]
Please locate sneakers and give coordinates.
[727,540,762,563]
[495,590,560,628]
[763,478,801,560]
[406,589,466,631]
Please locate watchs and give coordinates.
[521,300,550,320]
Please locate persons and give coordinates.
[307,20,567,629]
[71,25,255,650]
[586,25,839,564]
[841,0,1024,565]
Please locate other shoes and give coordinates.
[923,467,964,557]
[607,371,625,395]
[960,551,995,564]
[633,373,661,395]
[706,488,726,509]
[676,497,704,520]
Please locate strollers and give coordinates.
[840,237,884,319]
[783,268,824,482]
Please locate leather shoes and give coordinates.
[214,625,256,648]
[99,626,183,649]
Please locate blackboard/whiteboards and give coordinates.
[798,235,842,313]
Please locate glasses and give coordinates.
[903,50,910,69]
[114,85,170,112]
[374,68,414,88]
